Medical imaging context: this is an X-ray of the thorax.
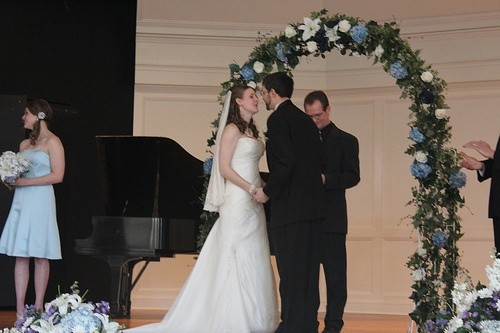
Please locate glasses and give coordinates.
[309,109,326,119]
[259,88,270,96]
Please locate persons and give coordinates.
[0,98,65,323]
[203,85,281,333]
[456,137,500,259]
[304,91,361,333]
[252,72,327,333]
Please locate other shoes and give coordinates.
[322,327,340,333]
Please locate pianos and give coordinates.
[73,135,219,320]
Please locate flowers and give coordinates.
[0,281,126,333]
[193,7,500,333]
[0,150,31,192]
